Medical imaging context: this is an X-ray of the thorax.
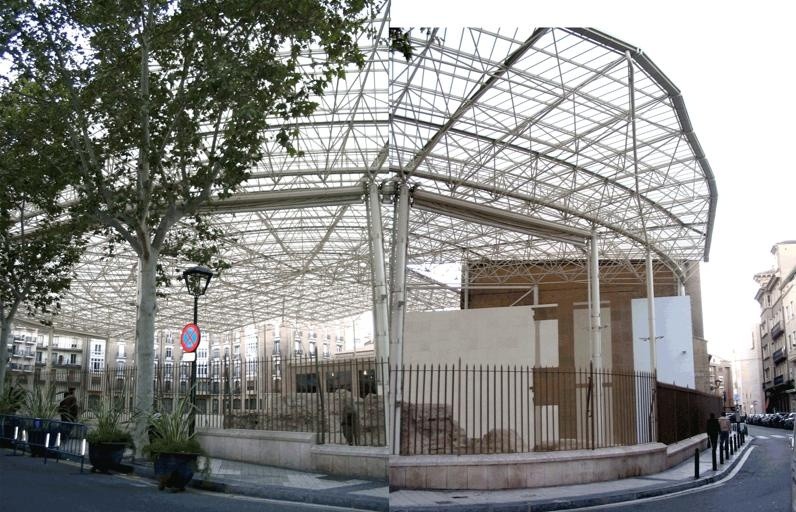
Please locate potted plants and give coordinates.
[0,371,212,492]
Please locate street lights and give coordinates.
[181,265,213,446]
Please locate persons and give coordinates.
[342,399,355,446]
[58,388,76,422]
[719,412,731,450]
[707,413,720,450]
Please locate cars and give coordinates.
[726,411,796,429]
